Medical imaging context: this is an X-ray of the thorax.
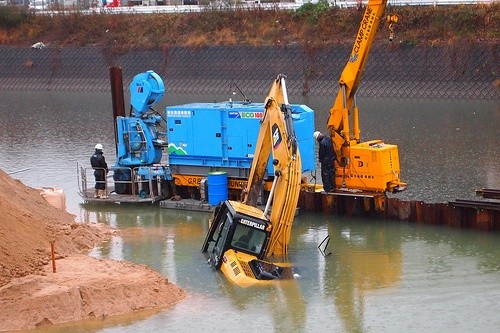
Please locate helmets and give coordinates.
[312,131,321,141]
[95,143,104,150]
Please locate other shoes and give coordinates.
[323,189,338,193]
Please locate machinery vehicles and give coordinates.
[200,73,302,290]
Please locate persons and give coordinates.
[313,131,336,193]
[90,143,108,199]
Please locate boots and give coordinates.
[99,190,109,199]
[94,189,101,198]
